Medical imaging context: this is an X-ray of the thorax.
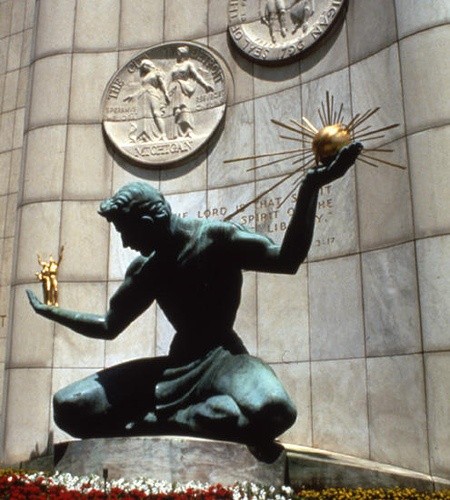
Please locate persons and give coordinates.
[23,142,366,444]
[35,244,65,307]
[258,0,315,44]
[122,46,215,141]
